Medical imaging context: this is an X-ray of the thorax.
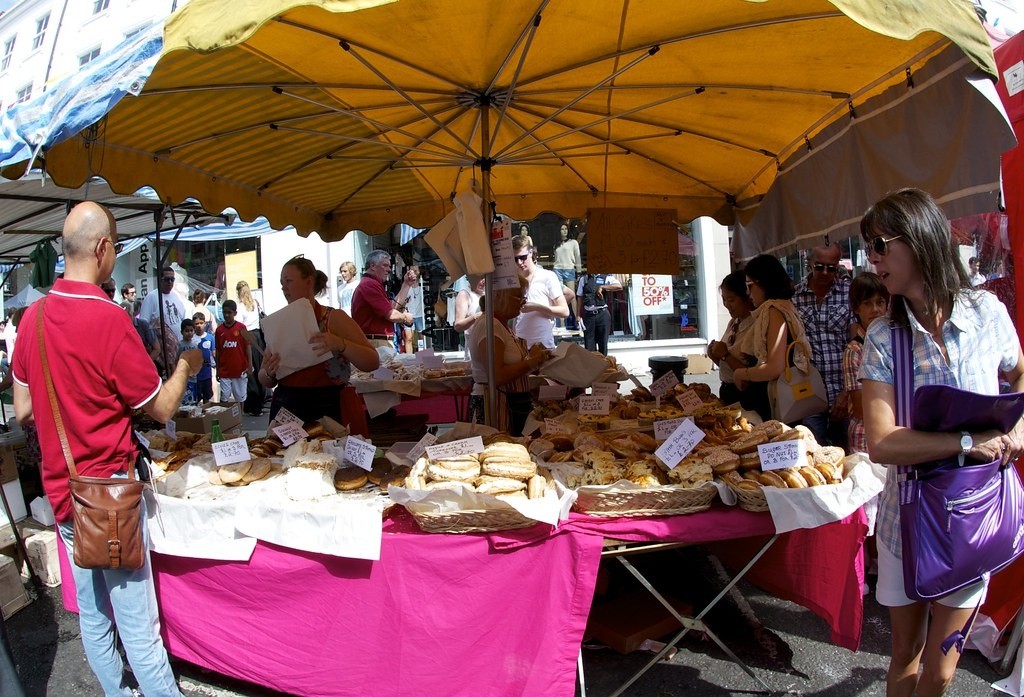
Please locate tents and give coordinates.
[0,168,238,380]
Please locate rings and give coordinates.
[269,366,270,369]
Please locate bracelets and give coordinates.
[267,373,276,378]
[403,315,406,323]
[722,352,729,361]
[338,339,347,353]
[746,368,749,380]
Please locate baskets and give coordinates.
[405,391,770,532]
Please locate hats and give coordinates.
[519,223,529,236]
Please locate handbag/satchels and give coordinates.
[340,385,368,441]
[255,299,268,346]
[768,339,829,425]
[69,475,145,571]
[897,385,1024,601]
[138,317,178,369]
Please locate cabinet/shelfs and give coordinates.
[661,285,698,326]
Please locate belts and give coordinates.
[365,334,394,340]
[584,308,607,315]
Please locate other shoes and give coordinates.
[240,410,263,417]
[574,321,581,330]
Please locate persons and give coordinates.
[856,189,1024,697]
[258,254,380,434]
[967,257,987,287]
[988,262,1003,280]
[0,223,615,417]
[707,269,771,422]
[841,272,892,603]
[732,256,814,428]
[467,275,551,437]
[790,242,858,447]
[11,200,204,697]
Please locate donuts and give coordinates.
[533,383,845,487]
[591,352,617,373]
[142,424,546,500]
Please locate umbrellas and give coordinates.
[0,0,1019,429]
[4,283,47,311]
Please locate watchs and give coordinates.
[958,431,973,466]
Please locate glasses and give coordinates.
[745,280,755,290]
[93,238,124,253]
[728,324,738,346]
[864,235,903,262]
[293,254,304,260]
[125,291,136,296]
[810,260,836,272]
[104,288,115,293]
[160,277,175,282]
[514,250,530,262]
[501,292,527,305]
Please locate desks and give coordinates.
[341,376,474,438]
[56,494,876,696]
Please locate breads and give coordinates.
[351,355,467,380]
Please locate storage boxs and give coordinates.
[0,444,65,622]
[172,400,242,437]
